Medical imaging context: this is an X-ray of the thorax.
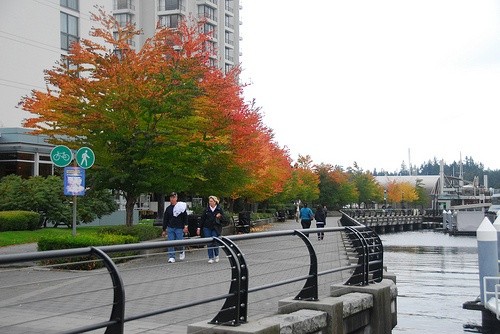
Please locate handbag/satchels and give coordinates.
[221,213,230,226]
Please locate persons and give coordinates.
[299,203,314,237]
[196,196,226,264]
[315,205,326,240]
[162,192,189,263]
[294,203,301,223]
[402,202,405,216]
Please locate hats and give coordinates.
[169,191,176,196]
[209,196,219,204]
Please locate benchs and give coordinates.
[232,216,256,234]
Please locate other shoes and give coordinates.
[215,254,219,262]
[320,237,323,240]
[168,258,175,262]
[208,259,214,263]
[179,252,185,260]
[318,237,320,240]
